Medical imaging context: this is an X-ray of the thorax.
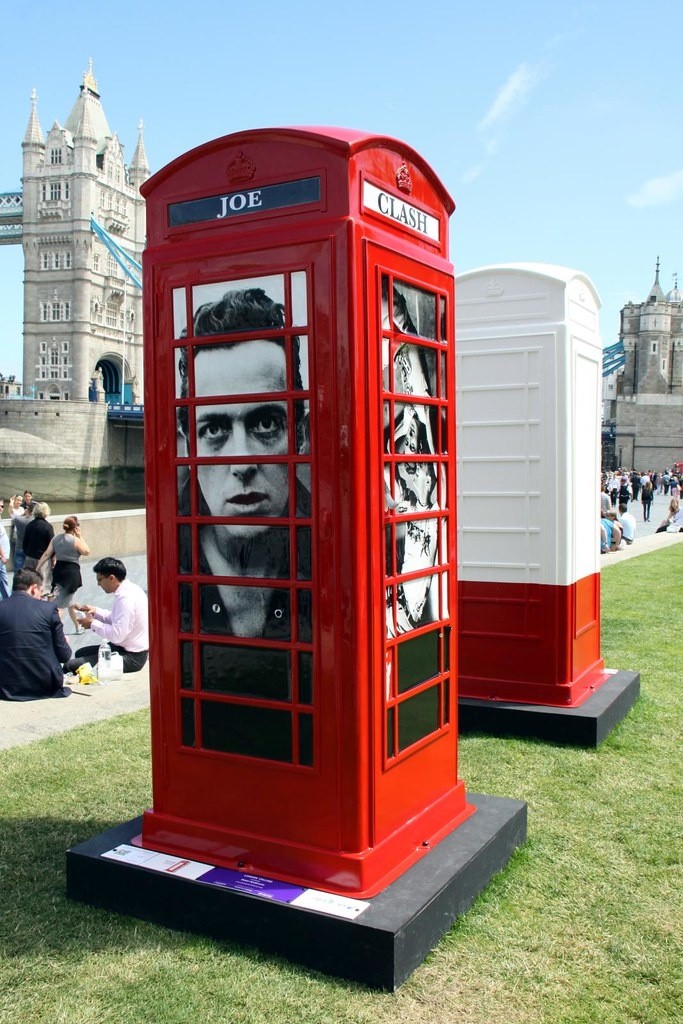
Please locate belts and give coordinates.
[622,536,632,541]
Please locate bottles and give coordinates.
[99,638,111,683]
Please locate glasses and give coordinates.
[97,575,109,582]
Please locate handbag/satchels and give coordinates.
[666,524,681,533]
[94,651,123,681]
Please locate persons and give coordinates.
[381,272,449,639]
[0,490,148,701]
[64,558,148,673]
[175,289,312,763]
[0,567,72,702]
[600,463,683,554]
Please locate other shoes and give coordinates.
[76,626,86,634]
